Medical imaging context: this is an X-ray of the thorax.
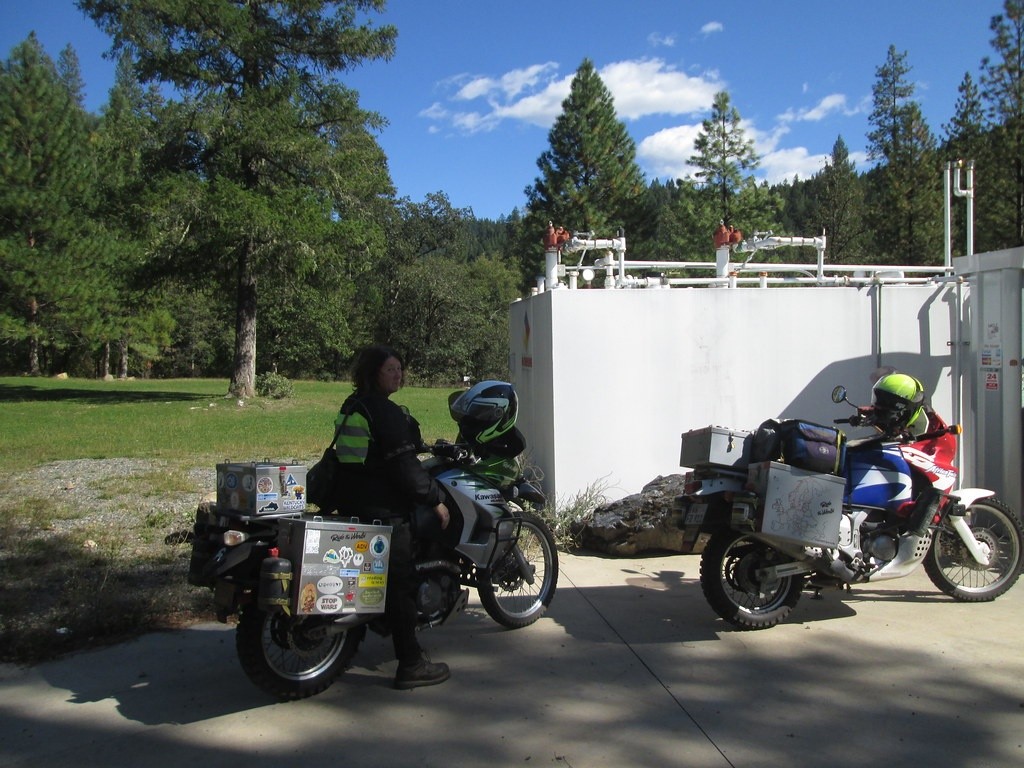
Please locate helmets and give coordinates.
[872,371,925,429]
[450,380,519,445]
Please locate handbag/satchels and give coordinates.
[306,449,373,514]
[780,417,848,475]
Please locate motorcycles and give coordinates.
[670,372,1024,630]
[165,379,560,700]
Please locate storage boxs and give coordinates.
[276,516,393,614]
[680,425,755,469]
[186,501,233,588]
[214,456,306,514]
[746,462,848,546]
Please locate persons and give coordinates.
[334,344,451,689]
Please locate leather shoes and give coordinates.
[394,660,450,688]
